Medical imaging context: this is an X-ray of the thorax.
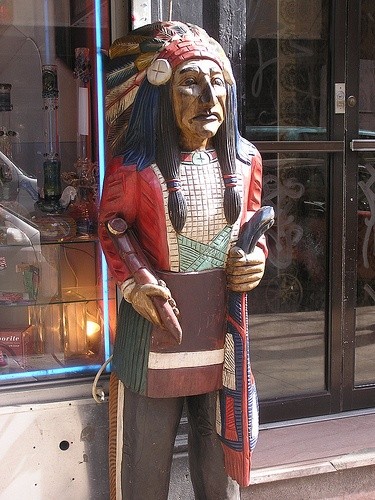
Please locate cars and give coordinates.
[0,203,58,298]
[247,125,375,312]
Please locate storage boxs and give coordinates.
[0,325,34,370]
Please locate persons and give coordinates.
[96,18,279,500]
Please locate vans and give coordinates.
[0,150,38,221]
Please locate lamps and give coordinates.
[84,320,101,336]
